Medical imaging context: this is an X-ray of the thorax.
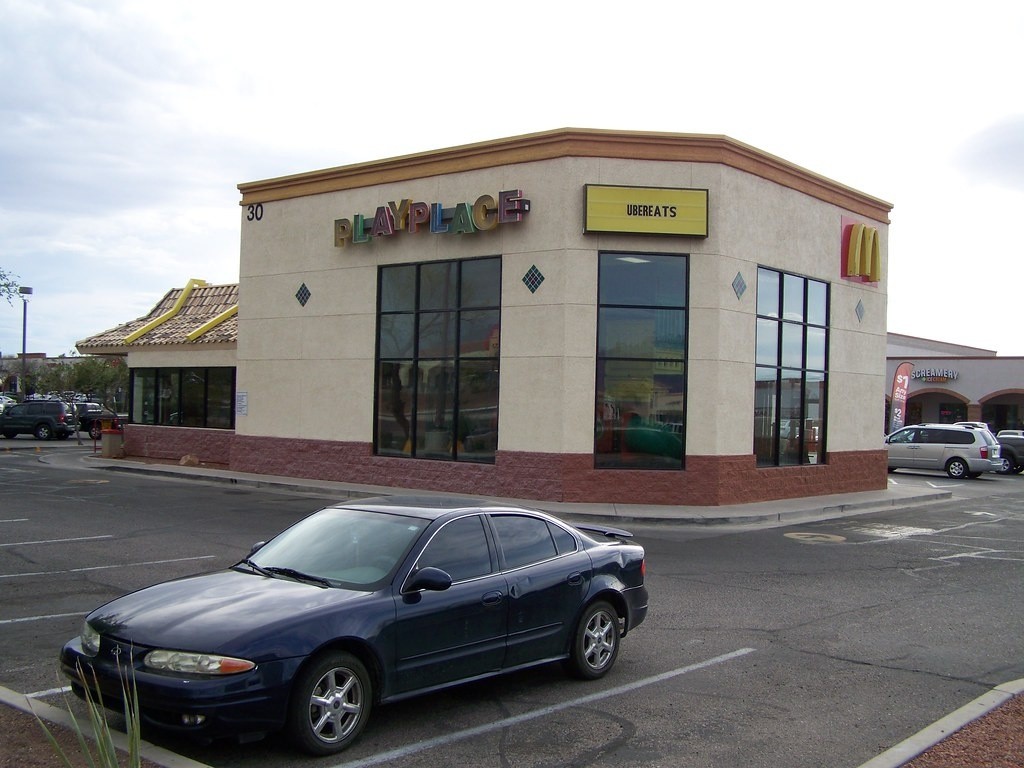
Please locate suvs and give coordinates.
[0,399,81,441]
[885,422,1004,479]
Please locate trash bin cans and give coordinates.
[100,429,121,457]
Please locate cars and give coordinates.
[58,493,654,758]
[780,419,801,439]
[0,396,17,408]
[26,390,109,402]
[955,420,1024,476]
[69,403,117,440]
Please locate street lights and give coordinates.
[18,286,33,400]
